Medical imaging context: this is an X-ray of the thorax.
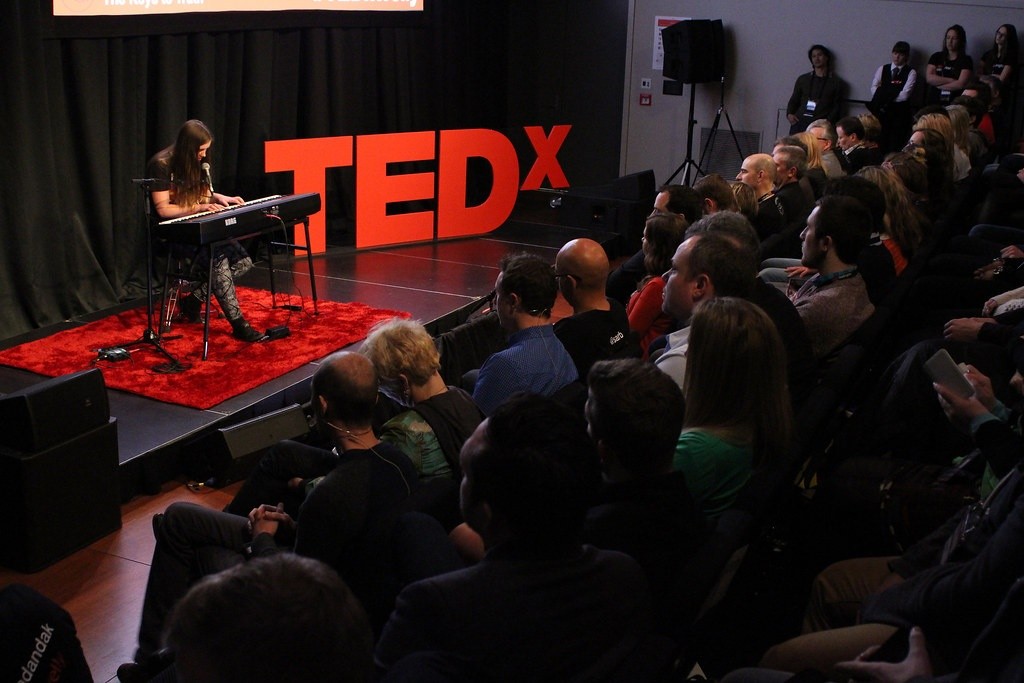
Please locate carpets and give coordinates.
[0,286,414,412]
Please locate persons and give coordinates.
[117,24,1024,683]
[144,119,264,342]
[0,566,94,683]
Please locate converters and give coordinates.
[266,327,292,339]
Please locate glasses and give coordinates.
[552,272,581,281]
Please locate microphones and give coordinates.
[308,415,319,428]
[201,163,214,197]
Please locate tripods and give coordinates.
[661,77,744,189]
[95,178,207,367]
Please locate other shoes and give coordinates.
[176,295,202,323]
[232,321,262,340]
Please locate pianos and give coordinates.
[152,190,322,362]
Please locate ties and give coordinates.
[893,67,900,78]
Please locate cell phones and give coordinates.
[924,349,974,399]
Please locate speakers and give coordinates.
[591,169,657,257]
[0,416,123,574]
[0,367,110,456]
[663,17,726,84]
[184,403,310,489]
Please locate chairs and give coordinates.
[742,382,841,509]
[670,520,748,623]
[605,632,679,683]
[856,306,892,347]
[903,247,931,281]
[880,271,916,311]
[151,203,207,333]
[826,342,866,387]
